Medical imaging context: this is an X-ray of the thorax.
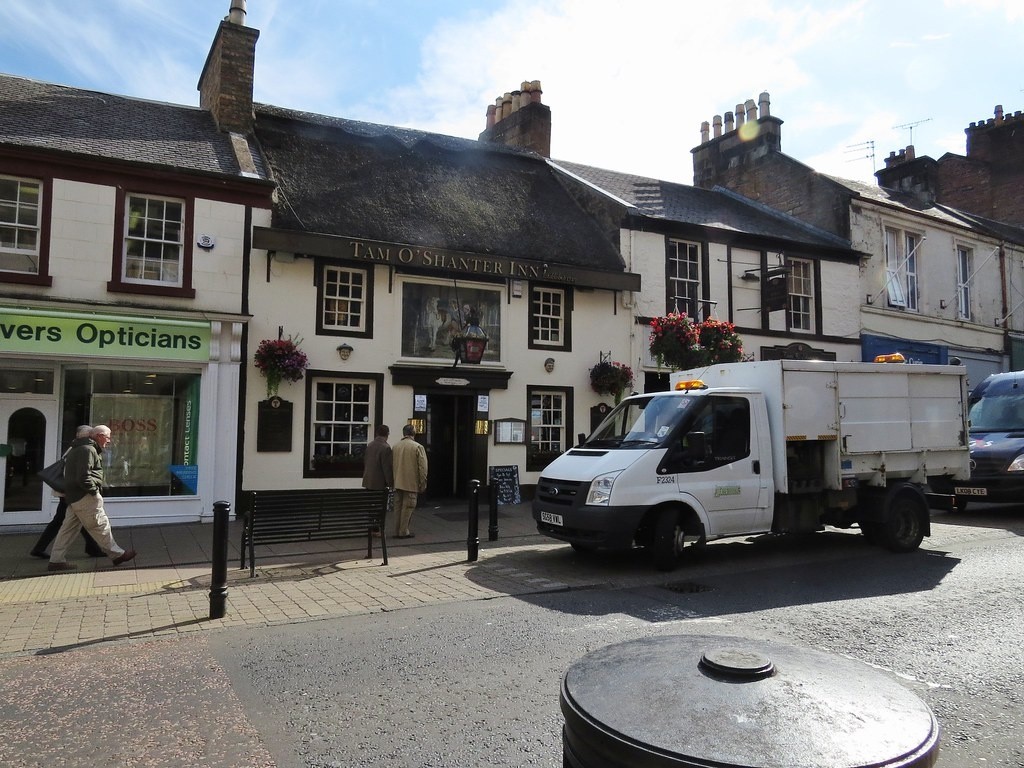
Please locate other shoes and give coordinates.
[400,533,415,538]
[89,551,108,557]
[393,535,400,538]
[31,551,50,559]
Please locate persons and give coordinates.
[389,424,429,540]
[28,426,109,560]
[46,422,137,573]
[361,425,394,540]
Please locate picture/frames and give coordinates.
[393,273,508,370]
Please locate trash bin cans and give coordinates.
[558,635,942,767]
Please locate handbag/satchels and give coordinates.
[37,447,67,494]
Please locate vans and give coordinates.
[941,371,1024,512]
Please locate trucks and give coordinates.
[530,353,972,571]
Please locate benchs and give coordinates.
[237,483,392,579]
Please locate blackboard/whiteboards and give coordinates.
[256,396,293,452]
[591,403,615,439]
[489,465,521,506]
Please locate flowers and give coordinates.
[588,359,634,405]
[253,332,310,398]
[651,304,701,370]
[697,305,745,366]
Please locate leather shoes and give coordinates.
[47,563,78,571]
[113,549,137,565]
